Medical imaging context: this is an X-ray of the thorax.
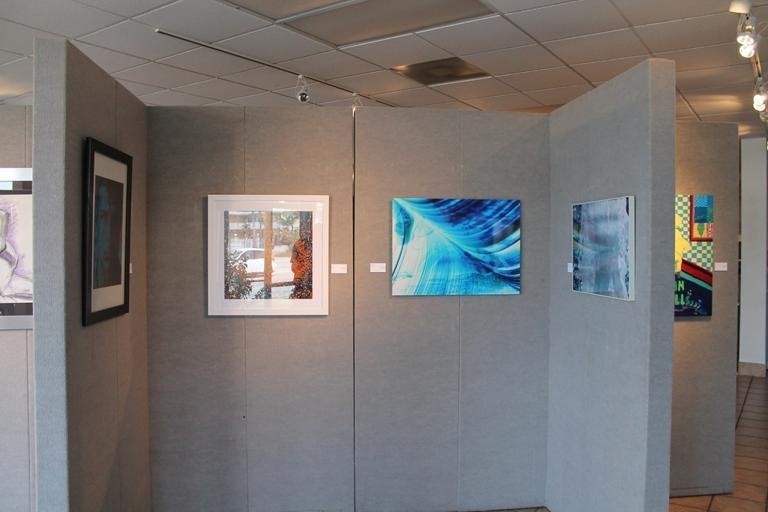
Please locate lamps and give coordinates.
[729,0,768,124]
[295,75,312,104]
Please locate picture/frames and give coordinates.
[570,196,634,302]
[0,168,36,333]
[83,136,135,328]
[204,193,331,318]
